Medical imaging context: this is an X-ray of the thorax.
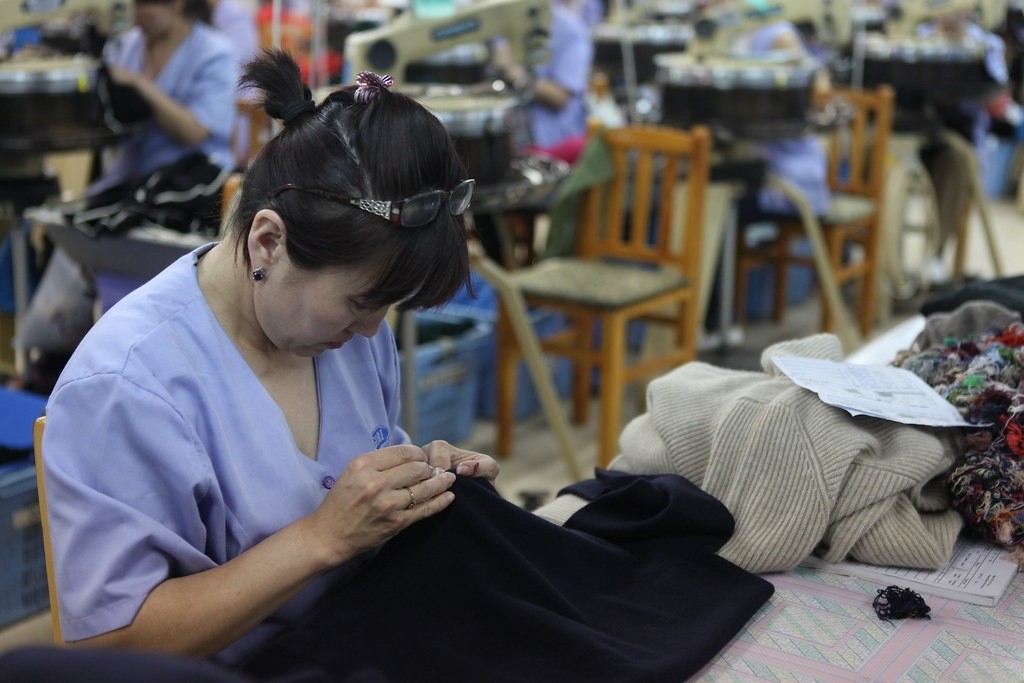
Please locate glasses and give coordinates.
[269,178,475,228]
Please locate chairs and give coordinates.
[493,118,711,472]
[736,82,891,344]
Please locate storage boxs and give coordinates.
[0,390,49,626]
[394,299,489,449]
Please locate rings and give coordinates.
[403,486,417,509]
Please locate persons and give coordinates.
[205,0,260,78]
[40,45,500,663]
[481,0,603,150]
[79,0,241,196]
[919,11,1009,156]
[734,20,836,219]
